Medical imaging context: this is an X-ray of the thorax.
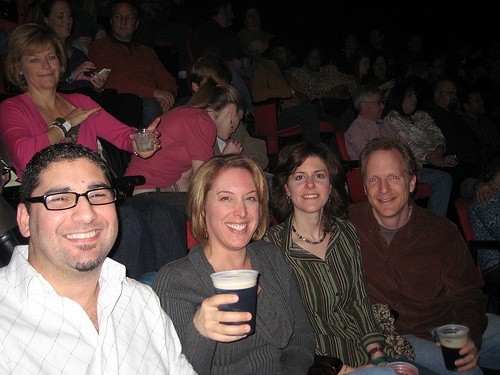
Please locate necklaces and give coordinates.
[292,221,326,245]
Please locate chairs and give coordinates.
[105,39,500,286]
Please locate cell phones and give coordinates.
[91,68,111,80]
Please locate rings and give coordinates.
[156,141,161,144]
[475,357,479,361]
[168,96,171,99]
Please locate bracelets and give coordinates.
[132,151,141,158]
[49,118,73,140]
[367,347,385,358]
[370,356,388,365]
[68,74,72,81]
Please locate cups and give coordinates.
[385,361,418,375]
[210,269,260,335]
[437,325,470,371]
[132,128,157,152]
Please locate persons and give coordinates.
[262,138,444,375]
[342,80,458,218]
[152,156,314,375]
[0,0,500,178]
[123,71,247,272]
[0,22,144,282]
[0,141,202,375]
[468,139,500,316]
[345,137,500,375]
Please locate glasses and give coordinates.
[26,187,117,211]
[363,99,385,105]
